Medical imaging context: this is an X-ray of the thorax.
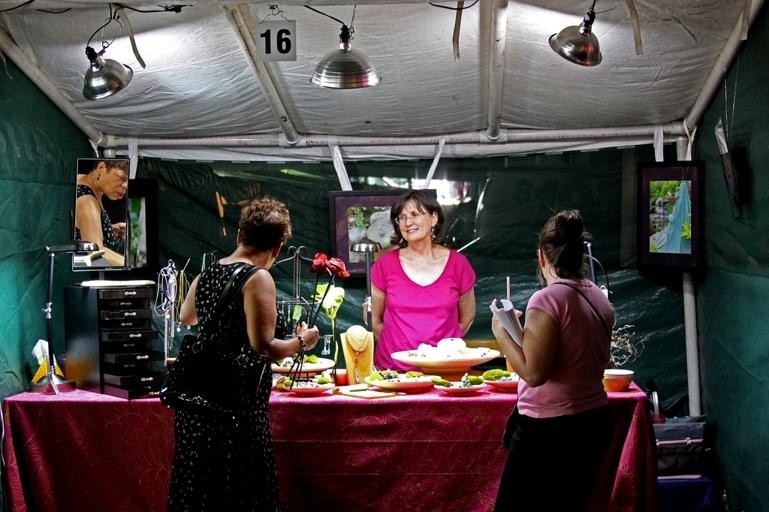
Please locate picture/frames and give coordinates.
[90,177,161,283]
[327,190,437,280]
[635,159,706,271]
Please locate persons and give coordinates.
[490,209,615,512]
[75,159,128,271]
[157,194,320,512]
[362,186,478,375]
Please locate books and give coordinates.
[489,296,523,347]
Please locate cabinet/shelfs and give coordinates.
[63,284,170,400]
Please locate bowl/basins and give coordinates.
[603,370,635,394]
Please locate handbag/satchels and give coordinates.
[159,334,280,431]
[502,406,520,451]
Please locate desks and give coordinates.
[3,373,648,511]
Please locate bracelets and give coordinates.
[298,336,308,352]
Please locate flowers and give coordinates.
[284,252,351,391]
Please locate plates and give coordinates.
[271,356,335,379]
[362,371,441,390]
[390,347,501,373]
[284,382,335,397]
[436,380,486,392]
[479,373,520,392]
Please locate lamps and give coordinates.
[303,5,382,91]
[80,5,133,101]
[29,241,101,395]
[548,1,602,66]
